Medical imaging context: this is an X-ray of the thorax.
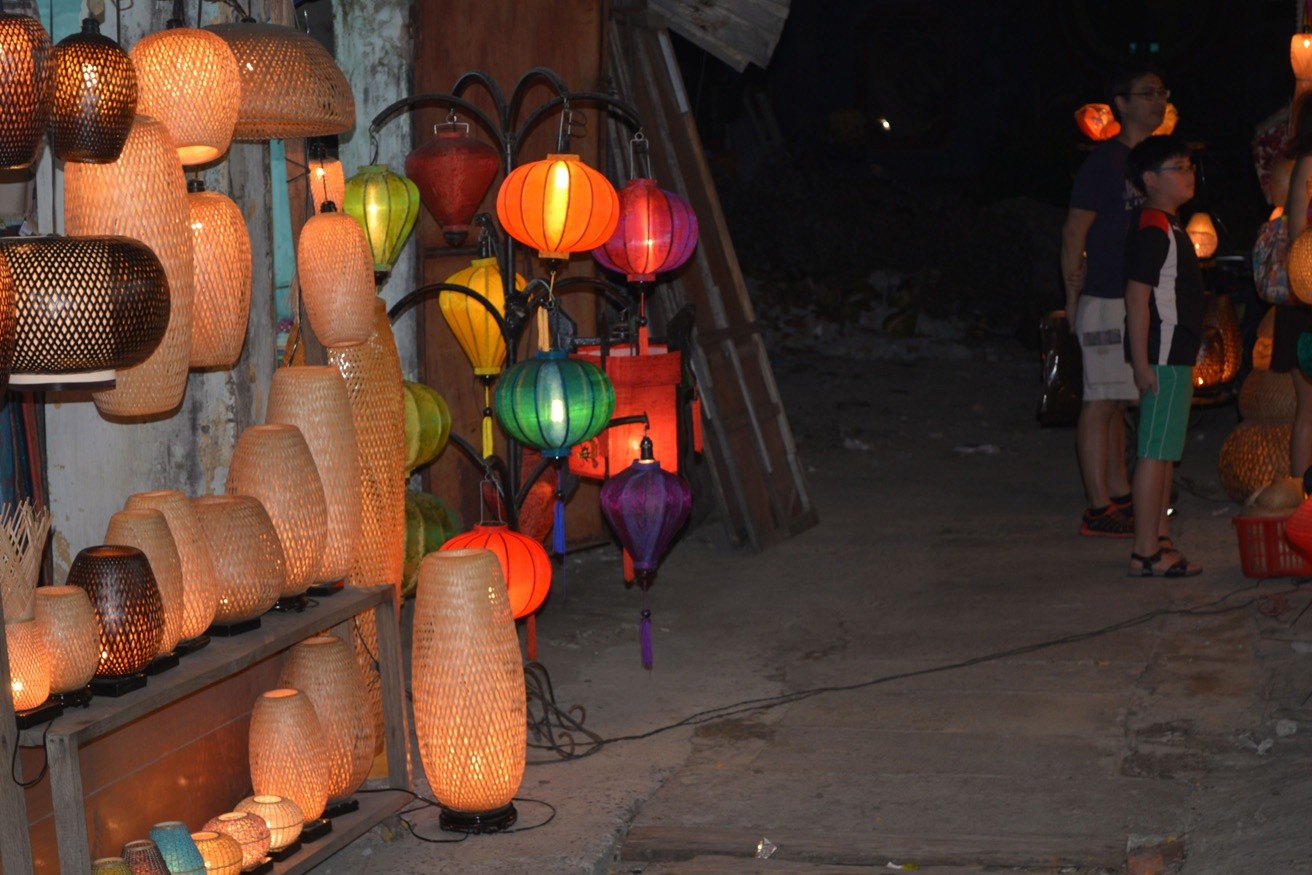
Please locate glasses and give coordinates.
[1120,87,1172,102]
[1146,160,1197,178]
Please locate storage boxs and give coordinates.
[1232,513,1312,577]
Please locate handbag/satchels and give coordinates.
[1250,213,1297,304]
[1036,312,1084,425]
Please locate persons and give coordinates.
[1059,60,1312,581]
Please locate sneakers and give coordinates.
[1113,496,1178,521]
[1079,506,1137,537]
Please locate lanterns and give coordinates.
[1291,33,1312,77]
[0,10,695,875]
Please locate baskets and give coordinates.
[1232,514,1312,579]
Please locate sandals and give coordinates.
[1128,547,1204,578]
[1156,536,1181,555]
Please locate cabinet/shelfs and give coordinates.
[0,582,415,874]
